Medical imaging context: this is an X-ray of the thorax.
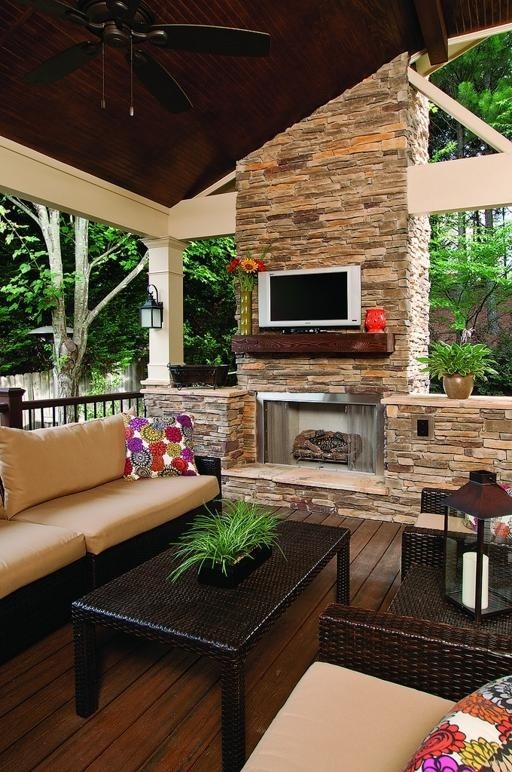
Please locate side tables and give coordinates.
[384,565,512,636]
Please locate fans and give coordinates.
[18,0,271,116]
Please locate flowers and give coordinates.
[225,239,273,291]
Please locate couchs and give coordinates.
[0,455,223,666]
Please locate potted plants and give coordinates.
[164,498,289,588]
[414,340,499,399]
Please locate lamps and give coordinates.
[140,284,164,329]
[441,469,512,619]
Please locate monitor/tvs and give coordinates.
[259,264,361,335]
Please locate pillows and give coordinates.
[0,406,139,521]
[401,675,512,772]
[461,482,512,545]
[0,493,7,520]
[121,411,200,481]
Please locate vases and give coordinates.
[365,307,388,333]
[239,291,252,335]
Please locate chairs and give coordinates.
[400,487,512,582]
[241,602,512,772]
